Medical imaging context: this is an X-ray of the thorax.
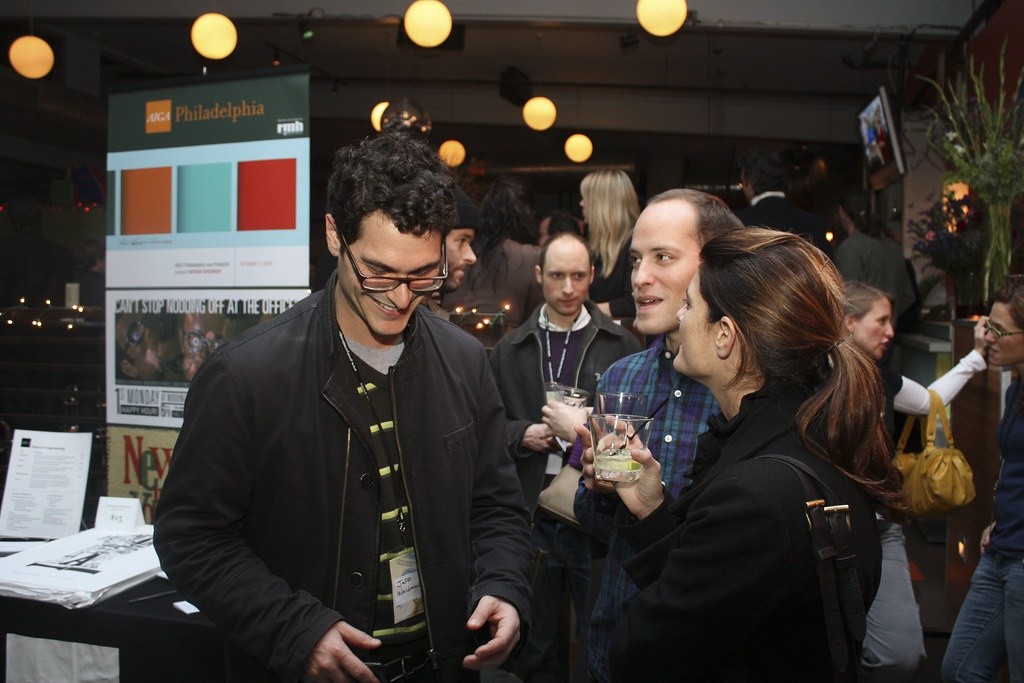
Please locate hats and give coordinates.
[451,184,480,228]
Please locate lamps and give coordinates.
[434,140,468,168]
[8,33,56,81]
[635,0,689,39]
[368,98,391,134]
[190,8,237,61]
[393,0,468,56]
[495,63,560,134]
[563,132,594,164]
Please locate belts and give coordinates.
[363,645,432,683]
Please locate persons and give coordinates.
[840,279,991,683]
[573,188,743,683]
[421,144,920,439]
[152,134,533,683]
[591,230,905,683]
[0,420,10,464]
[491,229,645,683]
[940,273,1024,683]
[0,195,105,307]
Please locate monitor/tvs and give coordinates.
[857,84,905,191]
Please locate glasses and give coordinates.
[985,319,1024,339]
[338,228,447,293]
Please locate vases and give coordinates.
[979,184,1015,307]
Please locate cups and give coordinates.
[596,391,648,418]
[554,386,589,410]
[586,413,654,484]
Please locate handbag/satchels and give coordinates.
[886,389,976,523]
